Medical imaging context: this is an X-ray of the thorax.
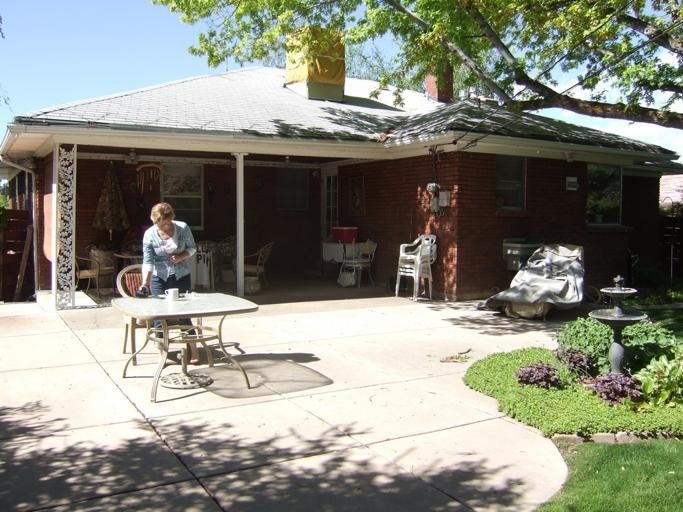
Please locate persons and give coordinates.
[136,202,202,367]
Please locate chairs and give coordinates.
[232,240,275,293]
[114,263,156,365]
[333,238,377,289]
[74,250,118,304]
[394,234,437,304]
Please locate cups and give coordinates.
[164,288,178,302]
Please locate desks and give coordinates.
[111,292,258,403]
